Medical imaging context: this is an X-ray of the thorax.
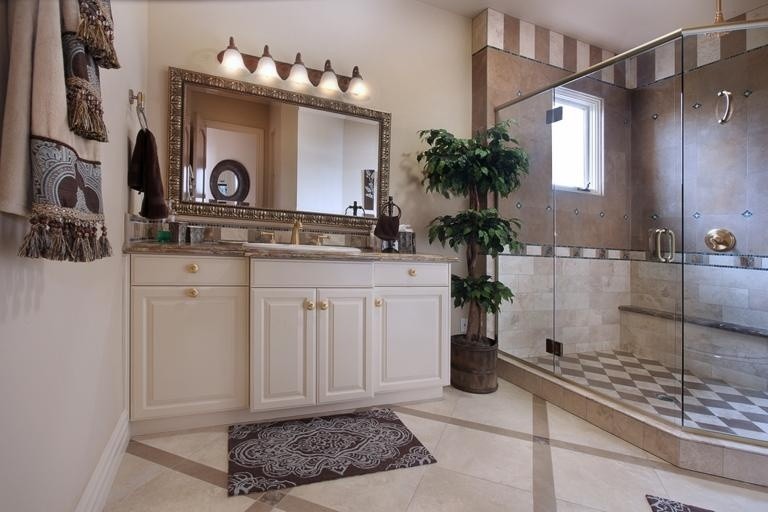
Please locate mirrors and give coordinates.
[208,159,251,206]
[168,64,395,235]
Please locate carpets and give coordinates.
[224,407,438,498]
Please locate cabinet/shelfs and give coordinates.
[373,264,446,396]
[250,287,374,410]
[131,254,249,423]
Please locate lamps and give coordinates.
[218,34,371,101]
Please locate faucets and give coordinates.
[291,221,303,244]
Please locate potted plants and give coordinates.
[416,118,526,393]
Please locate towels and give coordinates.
[127,128,172,221]
[375,215,402,239]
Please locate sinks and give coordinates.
[243,242,361,252]
